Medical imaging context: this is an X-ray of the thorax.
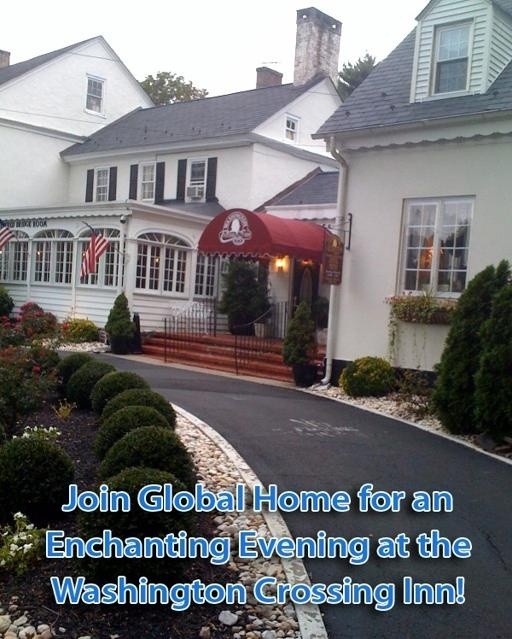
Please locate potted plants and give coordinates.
[104,289,137,356]
[215,256,276,339]
[281,301,320,387]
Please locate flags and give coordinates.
[0,220,14,251]
[80,228,109,278]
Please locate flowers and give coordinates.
[385,294,456,324]
